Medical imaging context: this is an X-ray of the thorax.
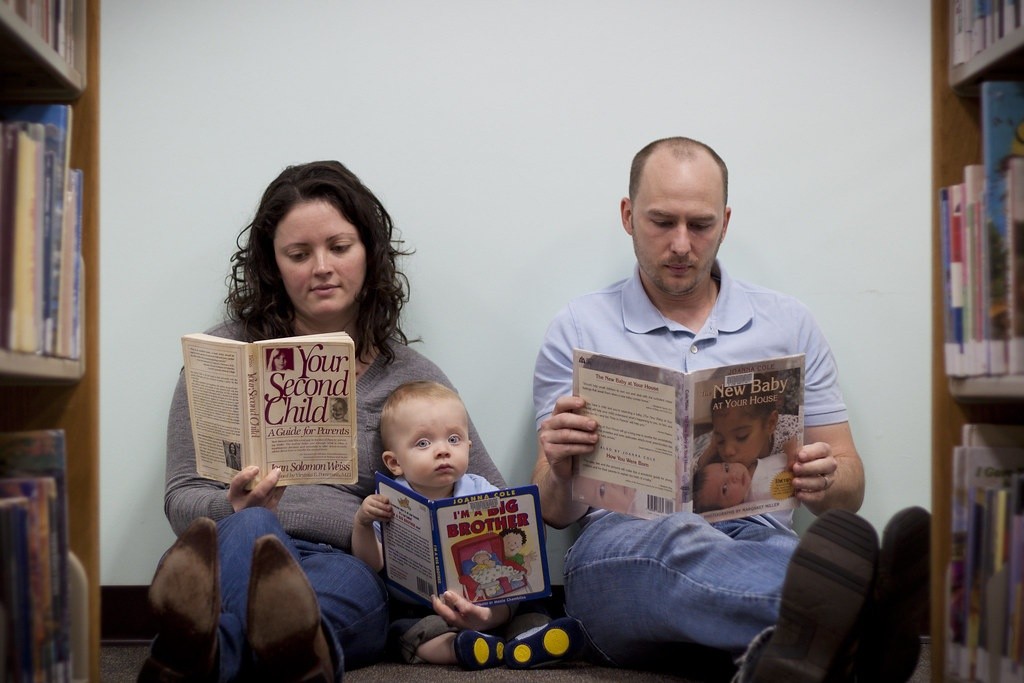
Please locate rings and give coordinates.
[824,476,830,489]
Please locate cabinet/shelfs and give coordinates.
[0,0,101,683]
[928,0,1023,680]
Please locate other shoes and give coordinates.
[747,510,880,683]
[860,507,933,683]
[243,535,334,683]
[503,616,586,670]
[456,630,506,668]
[139,516,221,683]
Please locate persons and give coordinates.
[225,443,240,470]
[331,398,348,422]
[268,348,291,370]
[573,464,639,514]
[132,161,516,683]
[349,382,584,668]
[533,140,933,683]
[692,373,798,513]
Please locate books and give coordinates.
[7,0,79,69]
[571,350,806,525]
[0,428,92,683]
[937,423,1024,682]
[374,470,553,613]
[0,98,88,361]
[938,79,1024,380]
[948,0,1024,66]
[180,331,359,492]
